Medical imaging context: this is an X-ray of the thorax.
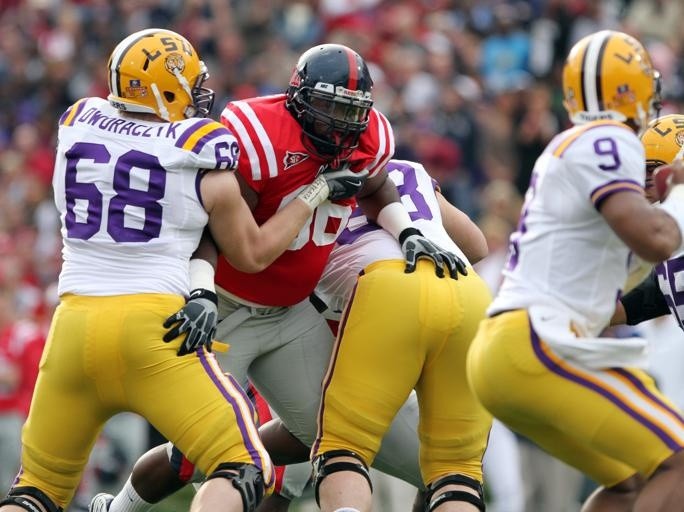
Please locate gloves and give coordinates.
[162,288,219,357]
[322,159,370,200]
[399,227,468,280]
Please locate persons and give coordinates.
[1,1,682,511]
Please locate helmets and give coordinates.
[562,29,663,128]
[637,113,684,167]
[107,27,215,123]
[284,43,374,162]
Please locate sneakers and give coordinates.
[87,493,114,512]
